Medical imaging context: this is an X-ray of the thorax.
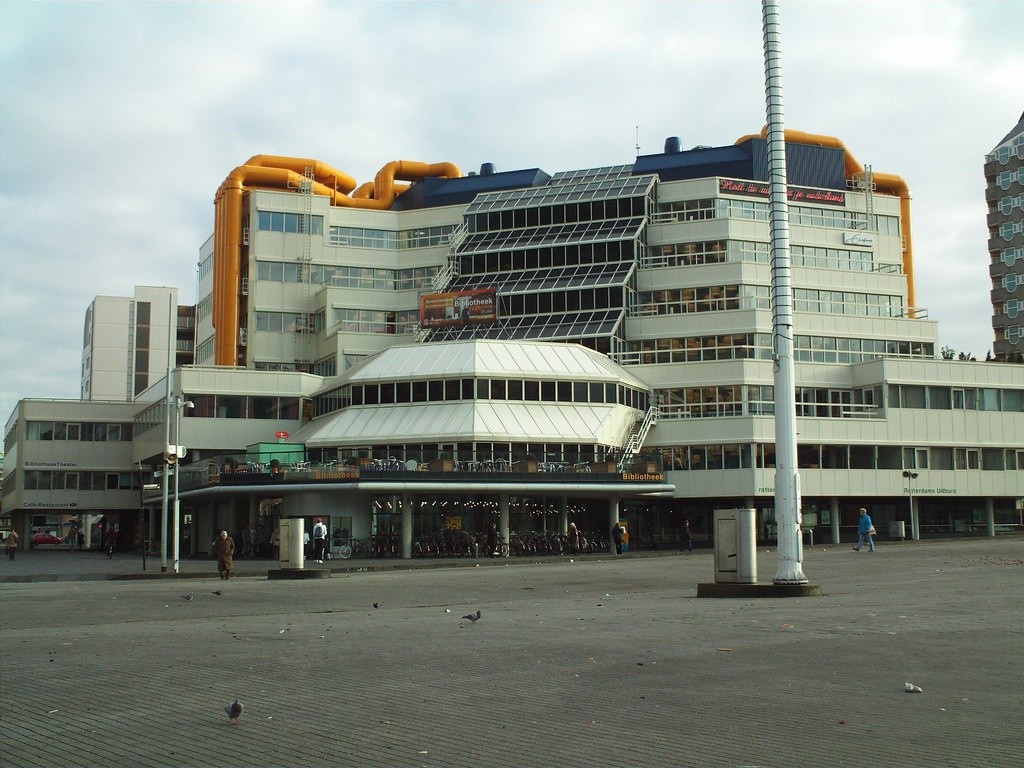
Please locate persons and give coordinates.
[104,527,116,556]
[214,523,281,560]
[70,527,84,549]
[214,531,235,580]
[6,528,19,561]
[302,517,329,564]
[852,508,875,553]
[440,518,694,559]
[462,305,471,327]
[764,523,771,542]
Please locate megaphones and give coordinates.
[165,451,177,464]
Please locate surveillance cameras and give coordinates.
[187,403,195,410]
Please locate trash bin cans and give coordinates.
[278,518,305,569]
[711,506,758,584]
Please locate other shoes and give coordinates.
[318,560,323,564]
[868,550,874,552]
[316,559,318,563]
[853,548,859,551]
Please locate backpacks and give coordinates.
[314,524,323,537]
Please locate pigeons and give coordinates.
[372,601,384,610]
[181,591,194,602]
[211,590,223,597]
[463,609,482,624]
[223,698,243,724]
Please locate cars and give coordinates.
[32,533,64,545]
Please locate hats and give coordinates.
[317,517,323,522]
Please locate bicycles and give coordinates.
[106,544,113,559]
[372,528,610,558]
[339,536,369,559]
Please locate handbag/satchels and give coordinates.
[5,547,9,556]
[868,524,878,536]
[14,533,19,543]
